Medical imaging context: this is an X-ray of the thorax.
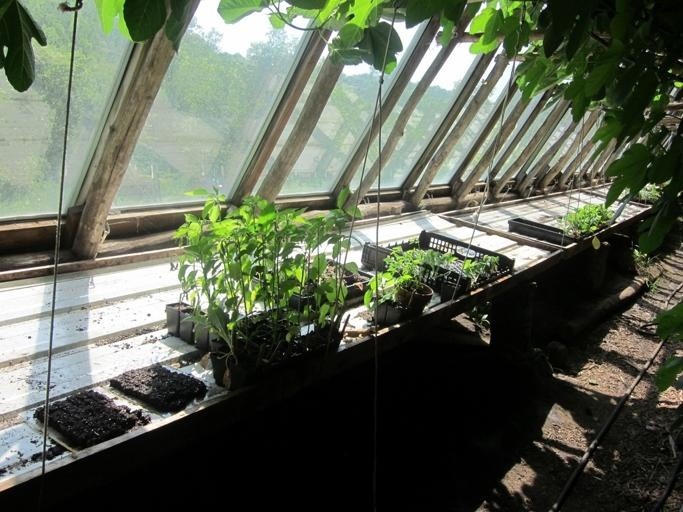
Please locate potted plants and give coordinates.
[364,272,413,325]
[165,185,375,391]
[384,245,499,317]
